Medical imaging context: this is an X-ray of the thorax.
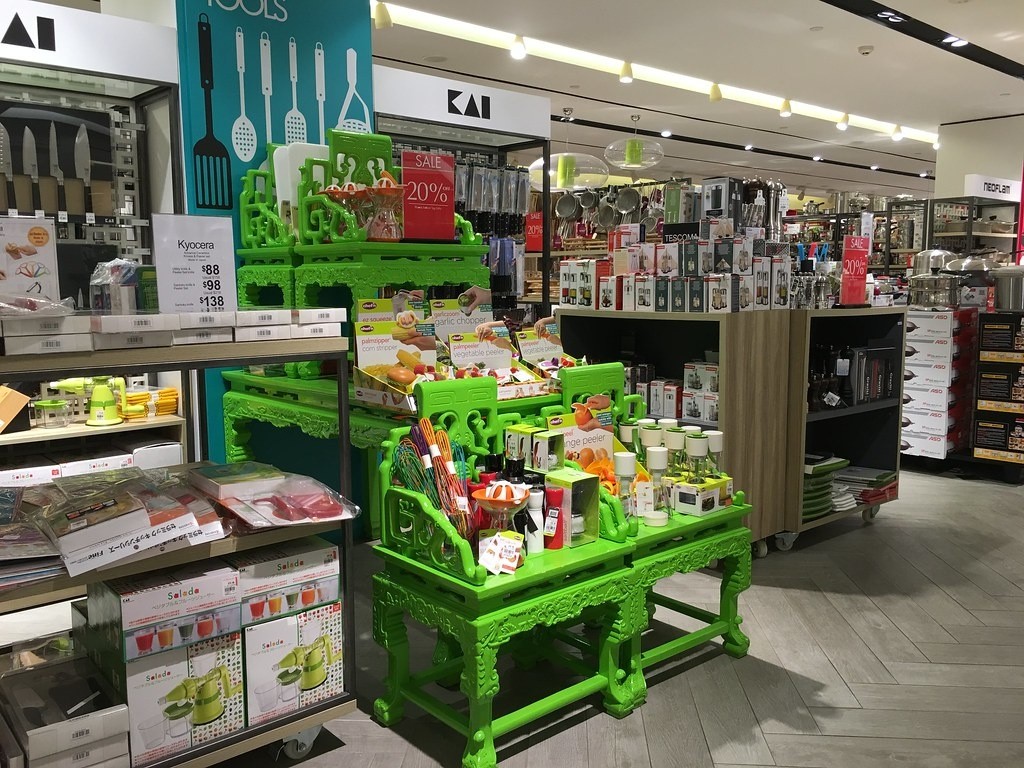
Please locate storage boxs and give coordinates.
[899,306,1024,464]
[223,535,346,728]
[559,176,792,312]
[86,557,246,768]
[2,653,130,768]
[1,307,348,355]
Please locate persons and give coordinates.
[534,315,562,346]
[457,285,492,314]
[394,309,436,351]
[474,319,511,350]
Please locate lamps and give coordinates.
[368,1,971,181]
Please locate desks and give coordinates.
[221,371,562,686]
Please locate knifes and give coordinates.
[452,170,528,237]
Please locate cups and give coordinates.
[248,579,331,622]
[134,607,233,655]
[254,680,280,712]
[137,714,169,749]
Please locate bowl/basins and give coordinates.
[32,400,69,429]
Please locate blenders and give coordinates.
[156,650,244,726]
[50,374,145,426]
[272,621,344,691]
[616,417,724,535]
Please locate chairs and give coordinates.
[371,377,633,768]
[235,141,299,377]
[530,361,753,706]
[293,128,491,378]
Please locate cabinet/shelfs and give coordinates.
[1,339,358,768]
[524,184,1023,313]
[554,306,909,560]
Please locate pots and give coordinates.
[276,668,303,701]
[555,186,639,239]
[907,243,1024,312]
[161,701,194,737]
